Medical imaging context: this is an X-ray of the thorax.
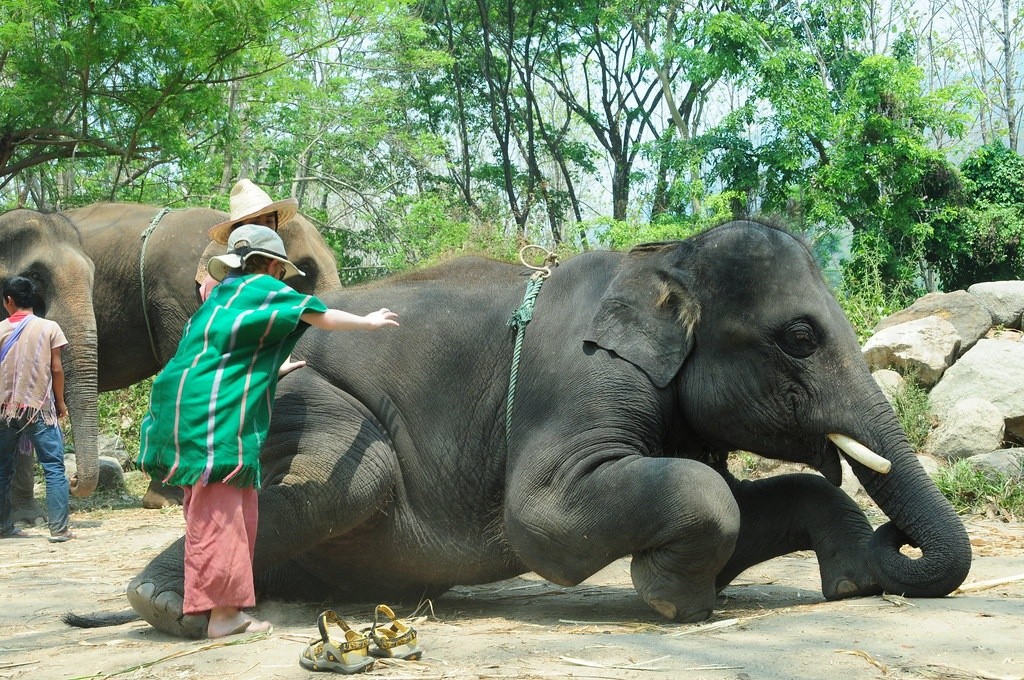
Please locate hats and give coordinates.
[207,178,298,245]
[206,224,306,280]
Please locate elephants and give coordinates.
[0,201,973,640]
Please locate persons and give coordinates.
[0,277,77,541]
[135,224,399,638]
[198,178,300,305]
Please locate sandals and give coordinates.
[299,609,373,673]
[358,605,423,659]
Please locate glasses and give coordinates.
[267,257,287,280]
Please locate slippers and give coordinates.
[47,531,77,543]
[0,526,30,538]
